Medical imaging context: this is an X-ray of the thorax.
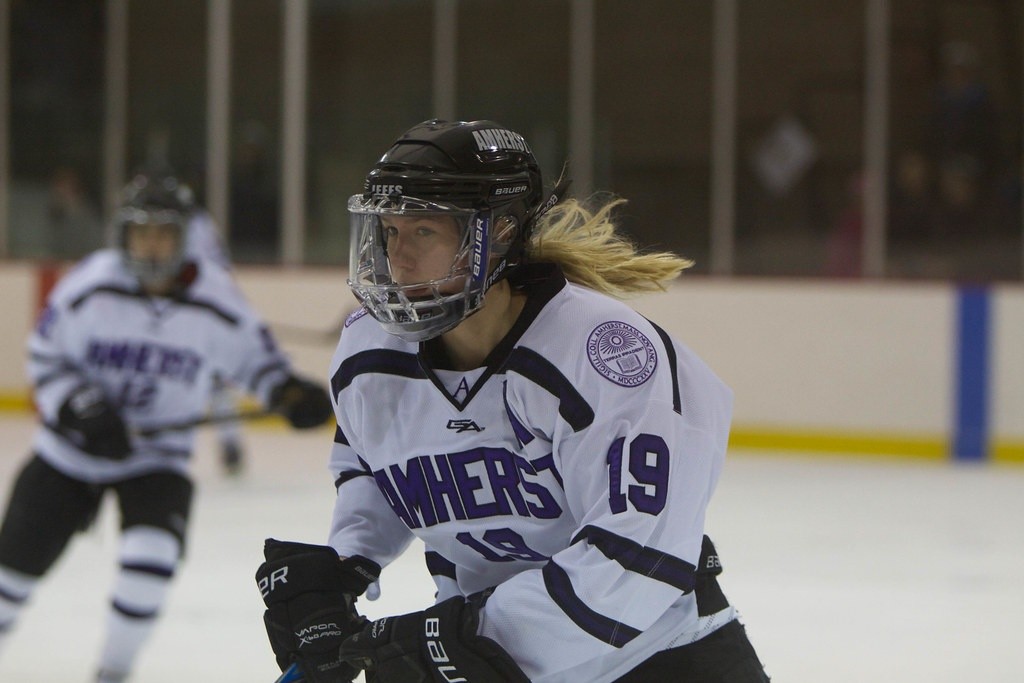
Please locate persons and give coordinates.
[0,169,334,683]
[253,119,770,683]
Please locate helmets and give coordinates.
[345,116,544,344]
[108,173,196,281]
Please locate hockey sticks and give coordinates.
[130,404,283,440]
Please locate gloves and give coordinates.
[254,539,361,683]
[59,386,134,461]
[273,377,332,430]
[339,586,531,683]
